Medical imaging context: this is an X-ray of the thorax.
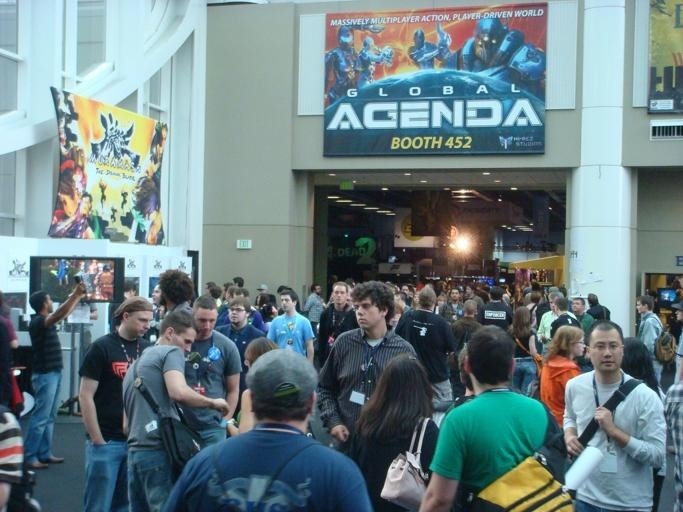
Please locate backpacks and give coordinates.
[647,316,678,363]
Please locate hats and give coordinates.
[113,296,153,318]
[246,349,317,407]
[671,300,683,310]
[257,283,268,290]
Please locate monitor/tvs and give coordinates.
[28,254,124,303]
[657,287,678,308]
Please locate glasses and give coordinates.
[227,308,247,313]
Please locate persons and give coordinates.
[0,267,683,512]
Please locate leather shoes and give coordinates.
[25,460,48,468]
[46,456,65,463]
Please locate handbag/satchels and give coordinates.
[381,417,429,512]
[159,417,207,468]
[472,451,576,512]
[533,353,545,378]
